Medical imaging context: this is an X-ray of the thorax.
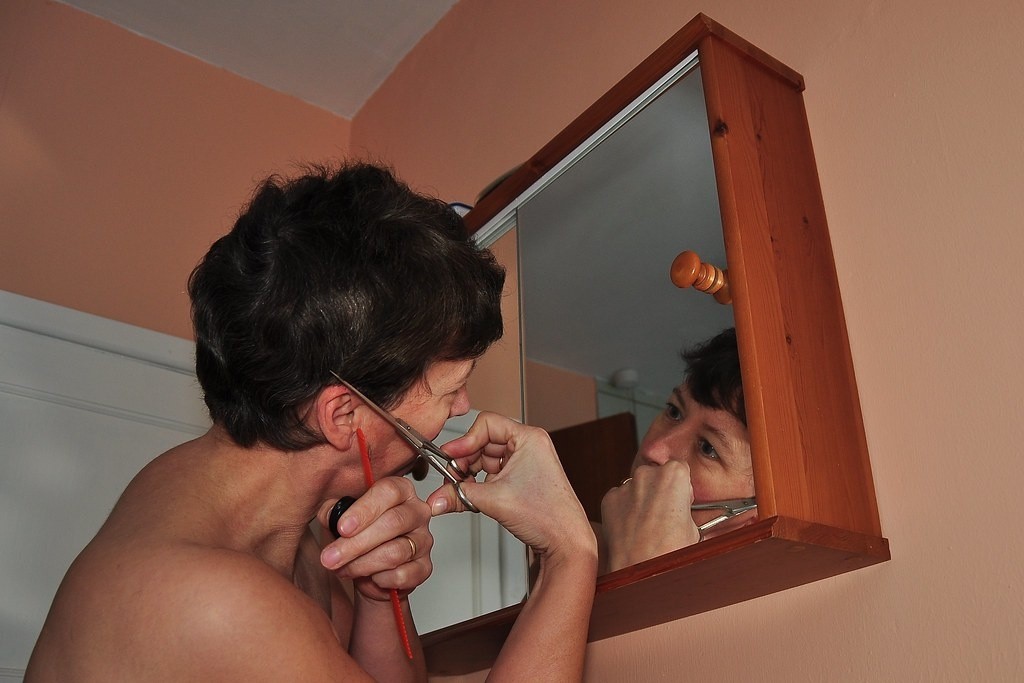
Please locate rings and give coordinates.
[403,536,416,560]
[622,477,632,485]
[499,457,503,471]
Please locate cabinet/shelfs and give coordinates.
[396,12,891,676]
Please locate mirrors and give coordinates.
[397,52,762,636]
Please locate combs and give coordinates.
[356,429,414,660]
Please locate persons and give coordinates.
[591,327,759,576]
[20,159,597,683]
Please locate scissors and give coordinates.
[619,476,758,543]
[326,366,481,513]
